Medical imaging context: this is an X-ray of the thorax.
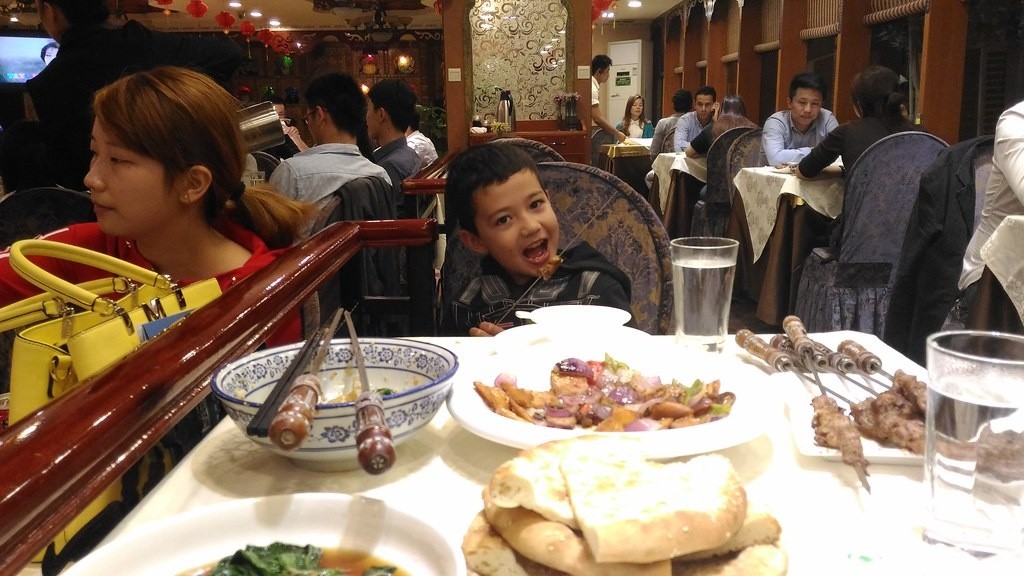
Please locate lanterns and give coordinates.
[154,0,285,54]
[591,0,612,21]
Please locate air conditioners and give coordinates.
[607,39,653,130]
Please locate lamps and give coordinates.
[344,0,413,42]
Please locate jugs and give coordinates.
[482,112,497,132]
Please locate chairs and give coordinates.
[309,175,400,337]
[442,137,674,336]
[0,185,98,395]
[660,127,674,153]
[252,152,280,182]
[794,131,995,369]
[591,128,614,168]
[690,126,765,302]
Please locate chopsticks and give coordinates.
[246,326,324,437]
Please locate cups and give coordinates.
[789,163,798,175]
[922,331,1024,561]
[670,237,740,355]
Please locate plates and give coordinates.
[516,305,632,326]
[779,371,924,466]
[497,324,651,336]
[773,170,792,175]
[447,337,787,450]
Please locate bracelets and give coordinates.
[839,166,847,177]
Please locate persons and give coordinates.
[366,78,423,295]
[41,42,60,65]
[958,101,1024,309]
[795,64,929,255]
[758,72,845,167]
[437,143,639,337]
[25,0,245,193]
[673,85,721,152]
[685,94,762,201]
[268,73,393,248]
[261,92,310,161]
[644,89,693,190]
[614,94,654,145]
[591,55,627,143]
[0,65,321,576]
[405,111,439,170]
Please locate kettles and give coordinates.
[497,91,515,131]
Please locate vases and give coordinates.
[556,97,580,131]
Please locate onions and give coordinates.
[492,356,729,431]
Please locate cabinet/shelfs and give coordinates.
[470,120,590,166]
[238,55,313,149]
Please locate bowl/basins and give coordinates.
[211,339,460,470]
[62,493,467,576]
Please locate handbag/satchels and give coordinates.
[1,239,226,574]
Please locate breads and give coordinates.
[461,435,788,576]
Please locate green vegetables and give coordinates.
[375,388,395,396]
[204,540,395,576]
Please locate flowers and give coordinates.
[553,92,581,104]
[490,121,511,138]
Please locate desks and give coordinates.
[651,151,707,239]
[732,166,845,325]
[979,215,1024,328]
[58,330,1024,575]
[598,145,652,204]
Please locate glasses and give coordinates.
[302,104,328,126]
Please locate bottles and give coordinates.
[473,115,480,127]
